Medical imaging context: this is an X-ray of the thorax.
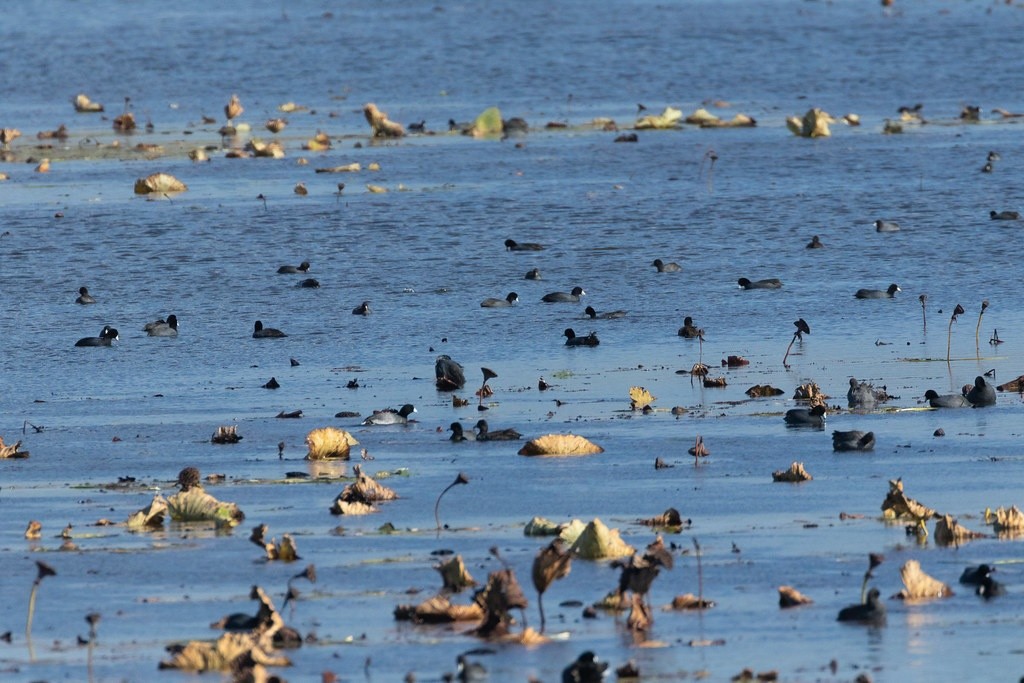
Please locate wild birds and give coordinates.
[141,315,180,338]
[738,277,783,291]
[446,419,524,441]
[782,378,887,453]
[989,211,1021,221]
[836,588,887,622]
[871,220,902,232]
[480,292,521,308]
[524,268,543,281]
[925,376,996,411]
[981,150,1000,173]
[75,286,96,305]
[959,563,1008,601]
[252,320,288,339]
[74,325,120,348]
[276,261,320,289]
[362,404,418,425]
[505,238,545,254]
[853,284,901,299]
[805,235,825,249]
[542,286,632,348]
[678,316,706,339]
[649,259,683,274]
[435,354,466,390]
[352,301,372,316]
